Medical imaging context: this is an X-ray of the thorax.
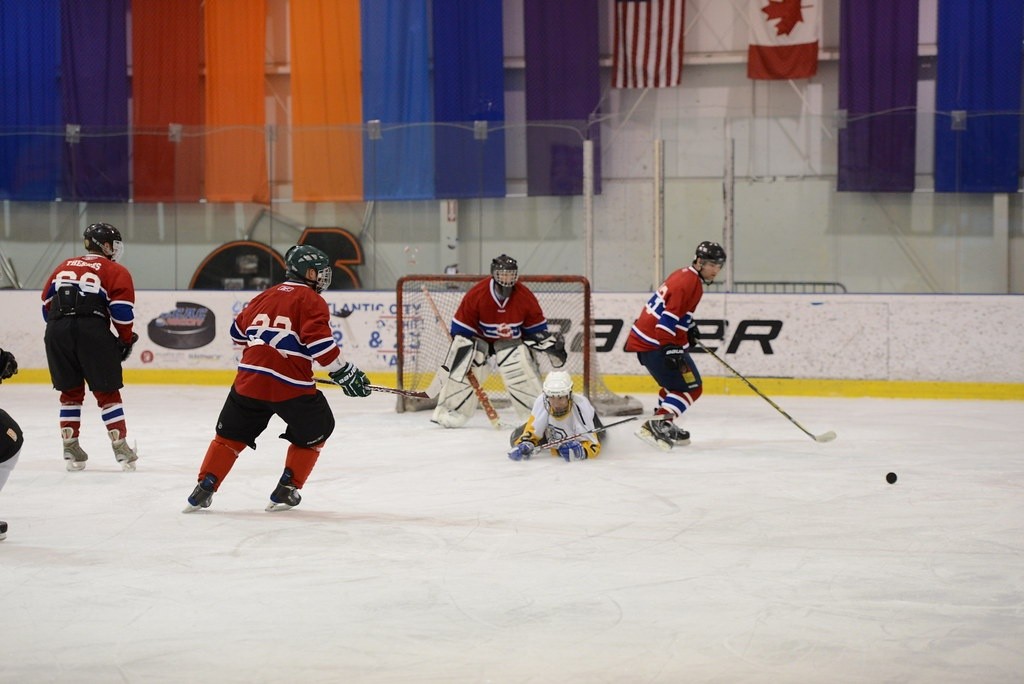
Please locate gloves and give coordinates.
[328,362,372,398]
[509,440,534,460]
[664,346,688,378]
[687,324,701,348]
[117,332,139,362]
[558,440,585,461]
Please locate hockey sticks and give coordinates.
[315,365,452,400]
[420,284,520,430]
[693,339,837,444]
[533,414,675,452]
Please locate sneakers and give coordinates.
[59,427,89,470]
[182,471,218,513]
[107,429,138,471]
[634,413,691,451]
[265,467,302,511]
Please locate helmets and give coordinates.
[82,222,125,262]
[284,245,333,295]
[491,253,520,296]
[542,370,573,416]
[696,241,727,263]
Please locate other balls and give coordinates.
[886,472,897,484]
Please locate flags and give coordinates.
[611,0,685,89]
[747,0,821,81]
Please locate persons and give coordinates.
[0,347,25,534]
[626,241,727,449]
[426,254,568,428]
[188,245,371,508]
[508,370,606,461]
[41,222,138,462]
[219,245,273,291]
